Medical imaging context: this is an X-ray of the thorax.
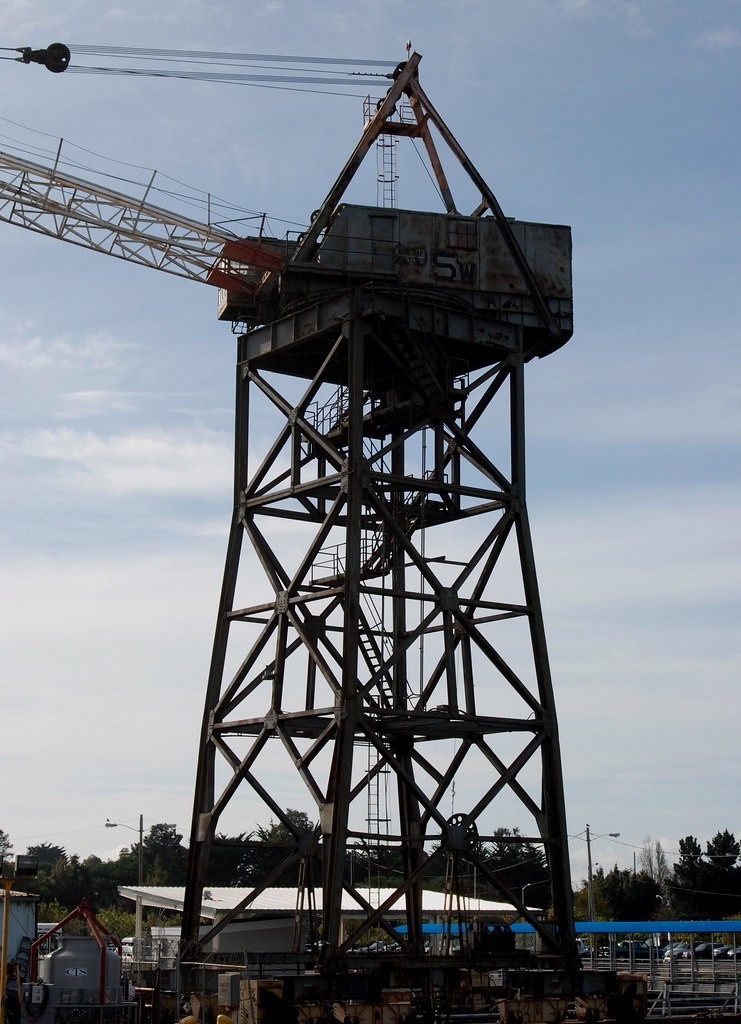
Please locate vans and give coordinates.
[120,937,146,957]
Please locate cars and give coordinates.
[664,942,699,960]
[682,943,724,960]
[576,937,669,963]
[712,944,737,960]
[655,942,681,960]
[727,946,741,959]
[360,941,431,953]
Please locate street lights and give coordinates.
[105,816,178,962]
[567,827,621,962]
